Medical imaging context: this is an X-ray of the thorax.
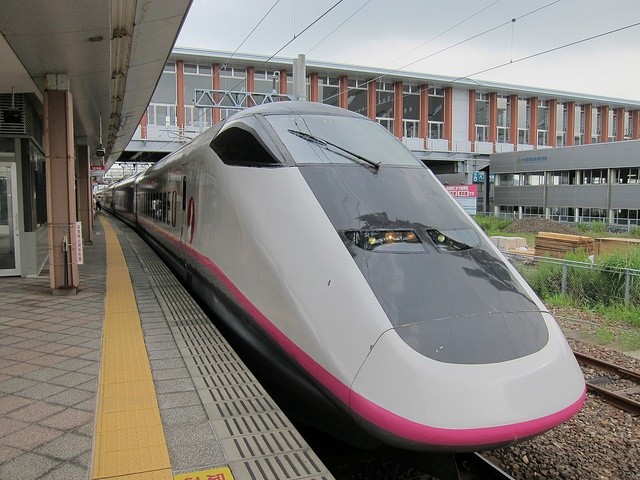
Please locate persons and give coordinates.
[96,196,102,210]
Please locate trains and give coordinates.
[96,100,587,453]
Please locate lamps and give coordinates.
[96,144,105,157]
